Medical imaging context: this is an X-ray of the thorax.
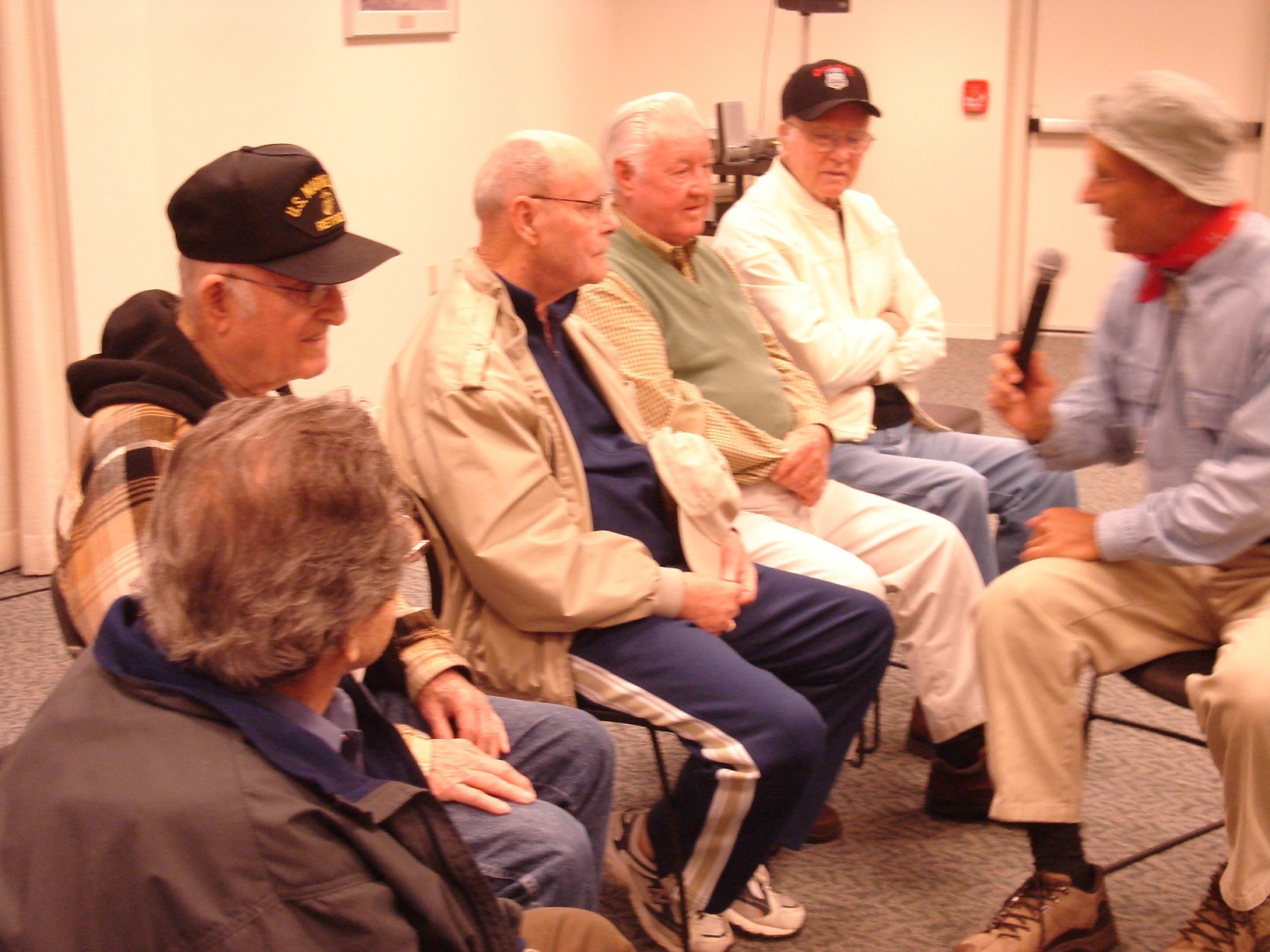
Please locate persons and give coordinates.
[49,141,619,917]
[715,57,1077,757]
[1,395,639,951]
[952,72,1270,952]
[371,130,898,951]
[566,87,988,842]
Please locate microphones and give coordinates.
[1011,246,1065,392]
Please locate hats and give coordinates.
[1080,67,1247,208]
[781,60,880,122]
[168,144,399,286]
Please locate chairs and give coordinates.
[407,488,690,952]
[1082,647,1223,876]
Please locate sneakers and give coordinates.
[1163,861,1269,952]
[721,864,806,938]
[604,807,735,952]
[803,805,841,842]
[924,750,993,818]
[953,863,1116,952]
[904,700,935,758]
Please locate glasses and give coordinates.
[219,273,326,307]
[531,191,614,219]
[787,121,874,154]
